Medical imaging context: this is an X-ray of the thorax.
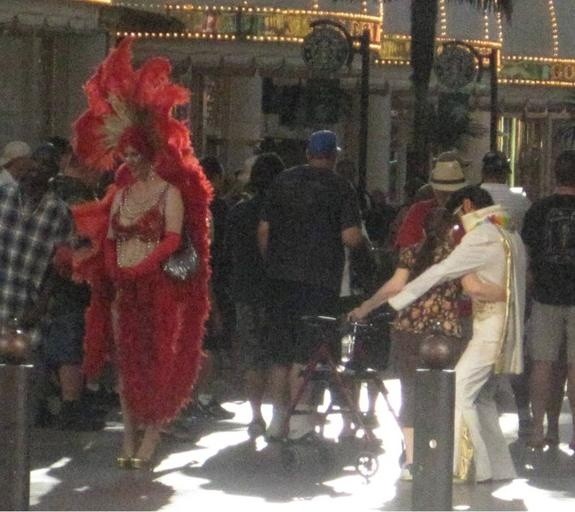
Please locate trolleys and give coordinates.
[275,305,414,478]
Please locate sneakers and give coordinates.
[247,404,378,442]
[163,396,235,440]
[32,383,120,431]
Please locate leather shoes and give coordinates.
[118,454,148,469]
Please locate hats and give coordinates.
[118,126,153,159]
[0,141,62,169]
[308,130,341,156]
[430,151,510,193]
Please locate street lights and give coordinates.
[430,33,498,193]
[300,13,370,217]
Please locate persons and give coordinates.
[0,125,575,484]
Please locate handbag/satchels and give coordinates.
[349,236,377,288]
[161,241,198,281]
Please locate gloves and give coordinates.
[104,233,182,287]
